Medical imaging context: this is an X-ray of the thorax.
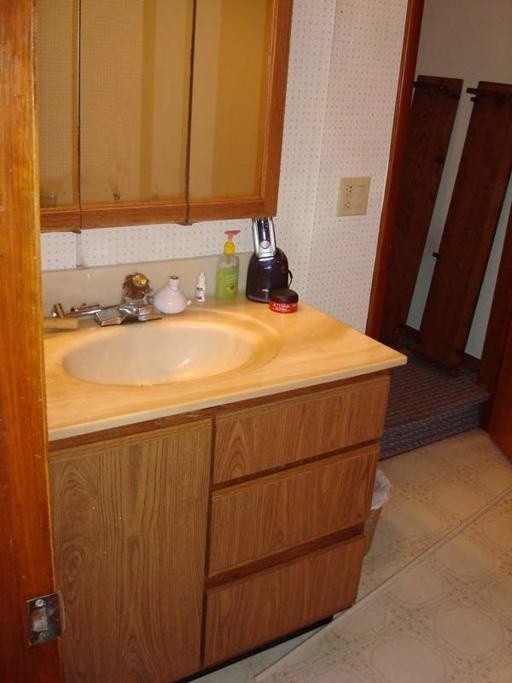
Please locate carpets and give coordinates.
[247,431,510,683]
[376,344,485,459]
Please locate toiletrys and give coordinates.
[215,229,240,300]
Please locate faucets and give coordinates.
[94,270,162,326]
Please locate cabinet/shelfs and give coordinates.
[42,415,211,683]
[203,373,390,671]
[34,0,295,233]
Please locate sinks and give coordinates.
[60,305,282,387]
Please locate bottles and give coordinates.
[196,274,208,301]
